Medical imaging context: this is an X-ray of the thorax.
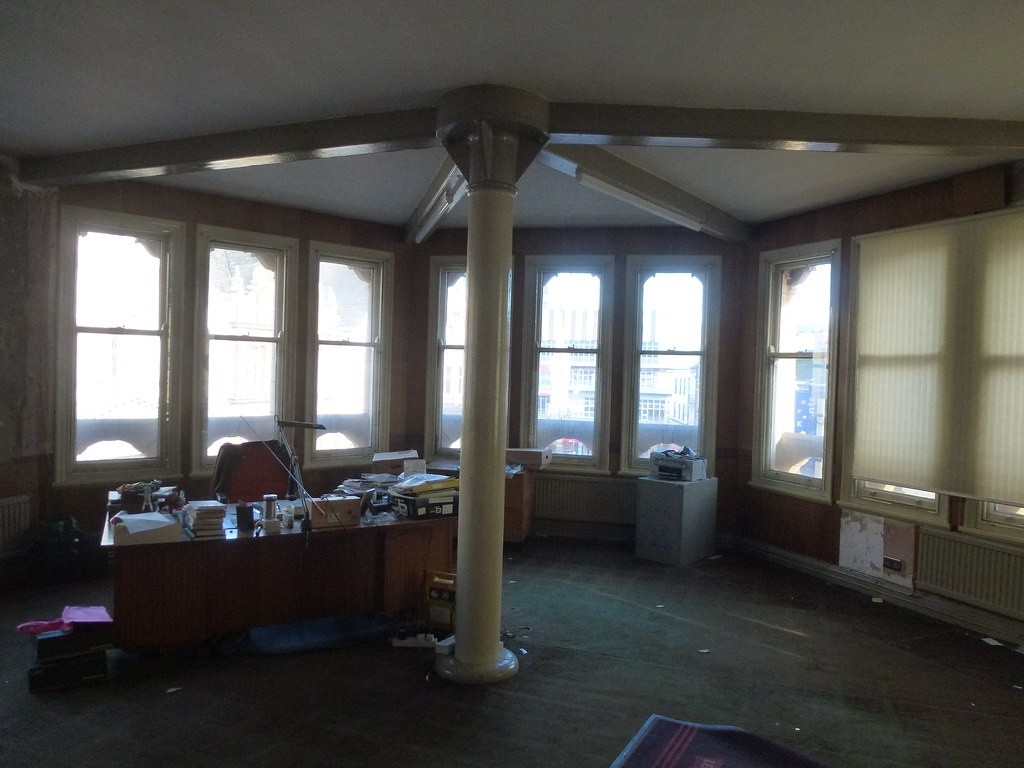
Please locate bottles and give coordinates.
[142,485,153,512]
[263,494,278,520]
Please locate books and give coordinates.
[182,500,228,542]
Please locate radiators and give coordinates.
[532,472,640,525]
[914,526,1024,621]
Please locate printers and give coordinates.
[649,452,707,482]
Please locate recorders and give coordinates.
[239,415,361,528]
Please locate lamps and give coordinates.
[415,193,455,245]
[574,170,704,233]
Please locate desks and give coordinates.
[98,498,456,657]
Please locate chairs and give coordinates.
[208,441,299,500]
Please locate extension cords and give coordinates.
[392,635,455,654]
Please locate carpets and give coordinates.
[607,714,825,768]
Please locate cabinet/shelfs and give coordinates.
[425,458,536,543]
